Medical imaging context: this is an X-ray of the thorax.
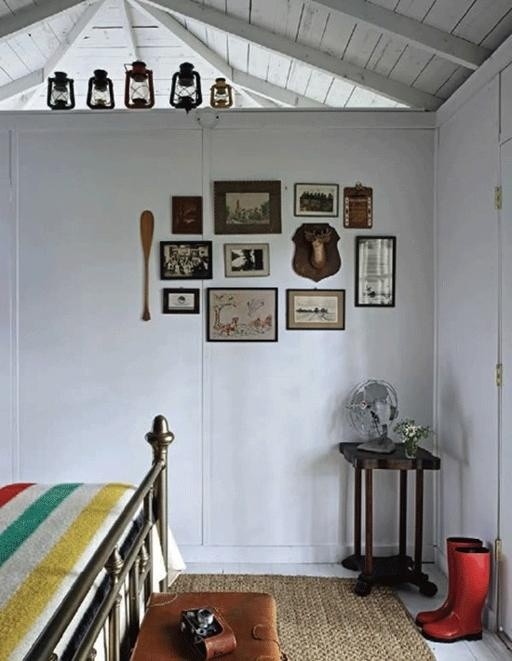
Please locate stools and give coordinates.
[337,441,441,598]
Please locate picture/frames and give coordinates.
[160,179,396,342]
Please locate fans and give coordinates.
[344,377,399,454]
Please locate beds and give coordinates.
[0,412,174,661]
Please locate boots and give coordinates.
[420,545,493,642]
[414,536,484,628]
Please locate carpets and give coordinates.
[164,571,435,661]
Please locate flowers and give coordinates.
[393,418,435,456]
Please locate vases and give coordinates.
[403,440,418,455]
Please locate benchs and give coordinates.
[129,592,280,660]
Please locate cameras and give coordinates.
[180,608,221,656]
